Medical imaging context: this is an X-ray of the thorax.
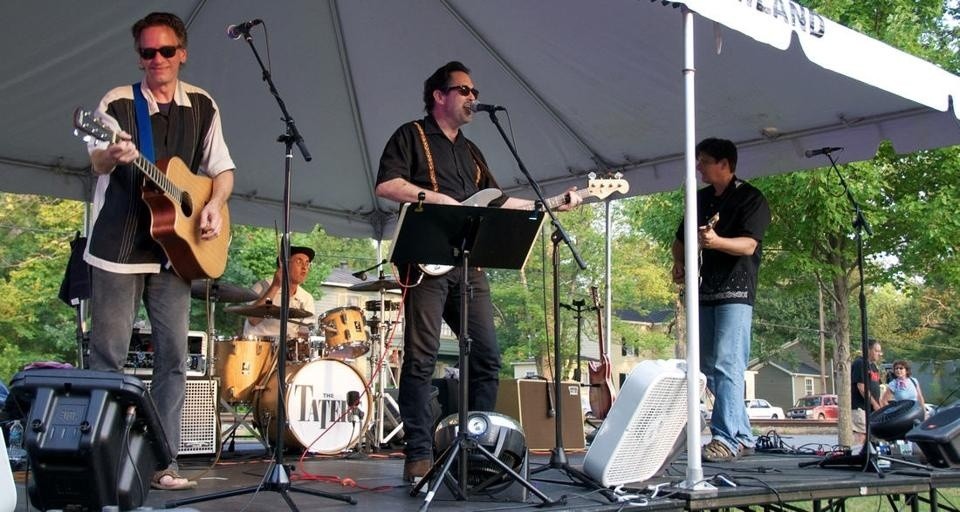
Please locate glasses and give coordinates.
[448,84,480,100]
[139,46,181,60]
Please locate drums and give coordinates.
[213,334,273,404]
[318,306,370,359]
[252,357,373,457]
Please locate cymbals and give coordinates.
[190,278,260,303]
[347,279,401,292]
[222,298,313,320]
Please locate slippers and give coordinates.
[150,469,197,490]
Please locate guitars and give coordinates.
[586,286,616,418]
[71,104,231,283]
[678,213,720,304]
[417,172,629,276]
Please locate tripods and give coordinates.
[491,113,617,503]
[164,38,359,512]
[799,156,933,478]
[410,252,554,512]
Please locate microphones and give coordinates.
[806,147,842,158]
[226,19,262,40]
[547,381,556,418]
[351,273,367,281]
[470,99,502,114]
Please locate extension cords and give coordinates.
[753,448,787,453]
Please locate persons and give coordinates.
[81,10,238,493]
[374,61,583,483]
[850,339,884,444]
[672,136,770,463]
[878,370,899,404]
[881,360,926,421]
[241,246,317,342]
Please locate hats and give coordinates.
[277,247,315,268]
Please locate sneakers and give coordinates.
[702,438,734,462]
[404,459,432,483]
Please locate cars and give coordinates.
[706,398,785,423]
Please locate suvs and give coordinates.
[786,394,840,422]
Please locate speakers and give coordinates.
[7,368,172,511]
[906,399,960,468]
